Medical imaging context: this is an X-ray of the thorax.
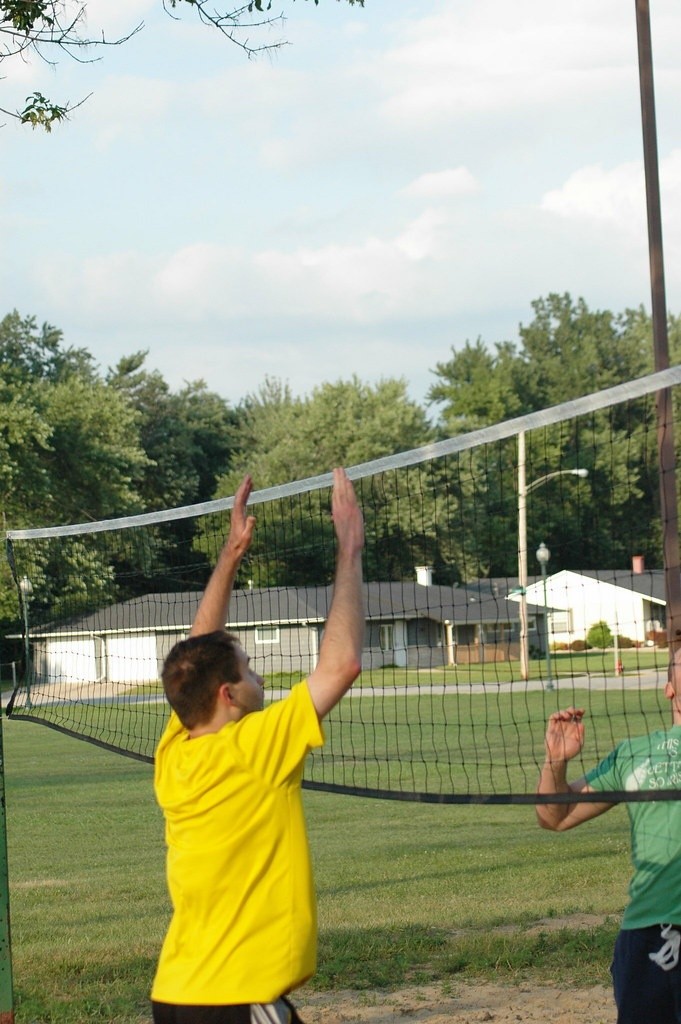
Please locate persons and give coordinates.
[150,466,364,1024]
[535,647,681,1024]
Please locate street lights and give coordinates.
[534,538,557,686]
[515,468,590,683]
